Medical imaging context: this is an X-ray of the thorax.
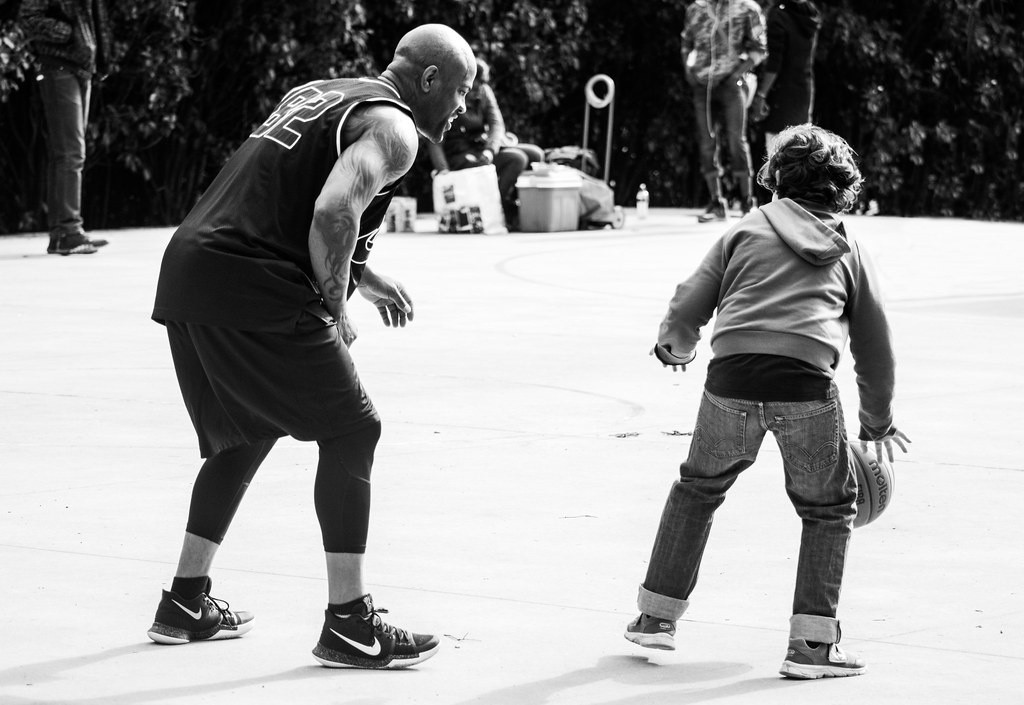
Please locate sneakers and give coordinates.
[312,593,440,670]
[779,636,868,678]
[623,611,675,650]
[148,588,254,644]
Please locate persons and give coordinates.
[18,0,118,253]
[430,57,544,214]
[681,1,822,222]
[622,122,912,678]
[151,24,476,670]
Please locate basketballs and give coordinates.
[848,435,896,529]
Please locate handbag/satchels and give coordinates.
[430,164,508,236]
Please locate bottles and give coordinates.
[636,183,650,219]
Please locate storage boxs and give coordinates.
[515,175,582,232]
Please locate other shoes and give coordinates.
[80,230,109,247]
[697,211,725,222]
[47,232,97,254]
